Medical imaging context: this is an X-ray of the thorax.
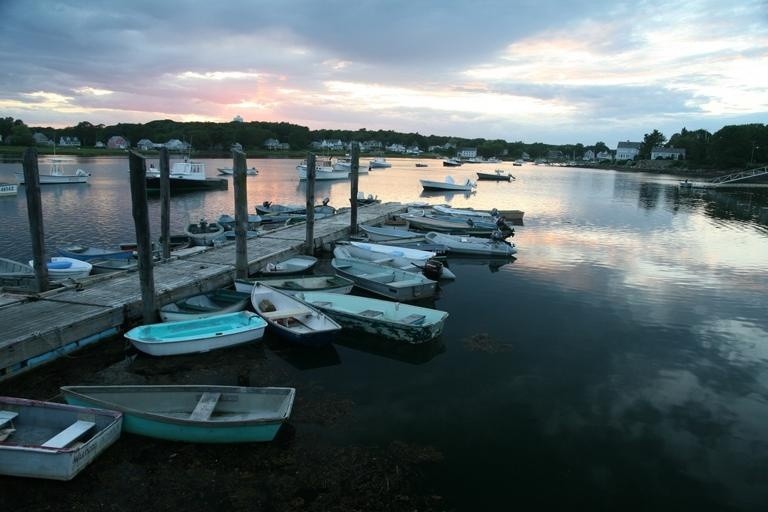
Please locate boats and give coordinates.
[419,188,476,202]
[418,176,478,192]
[369,159,392,170]
[416,163,429,167]
[298,159,349,181]
[476,169,515,183]
[465,156,502,164]
[512,162,522,167]
[1,180,19,200]
[514,159,523,164]
[336,153,369,174]
[216,165,259,177]
[125,158,227,197]
[443,160,460,167]
[19,157,89,188]
[679,178,692,187]
